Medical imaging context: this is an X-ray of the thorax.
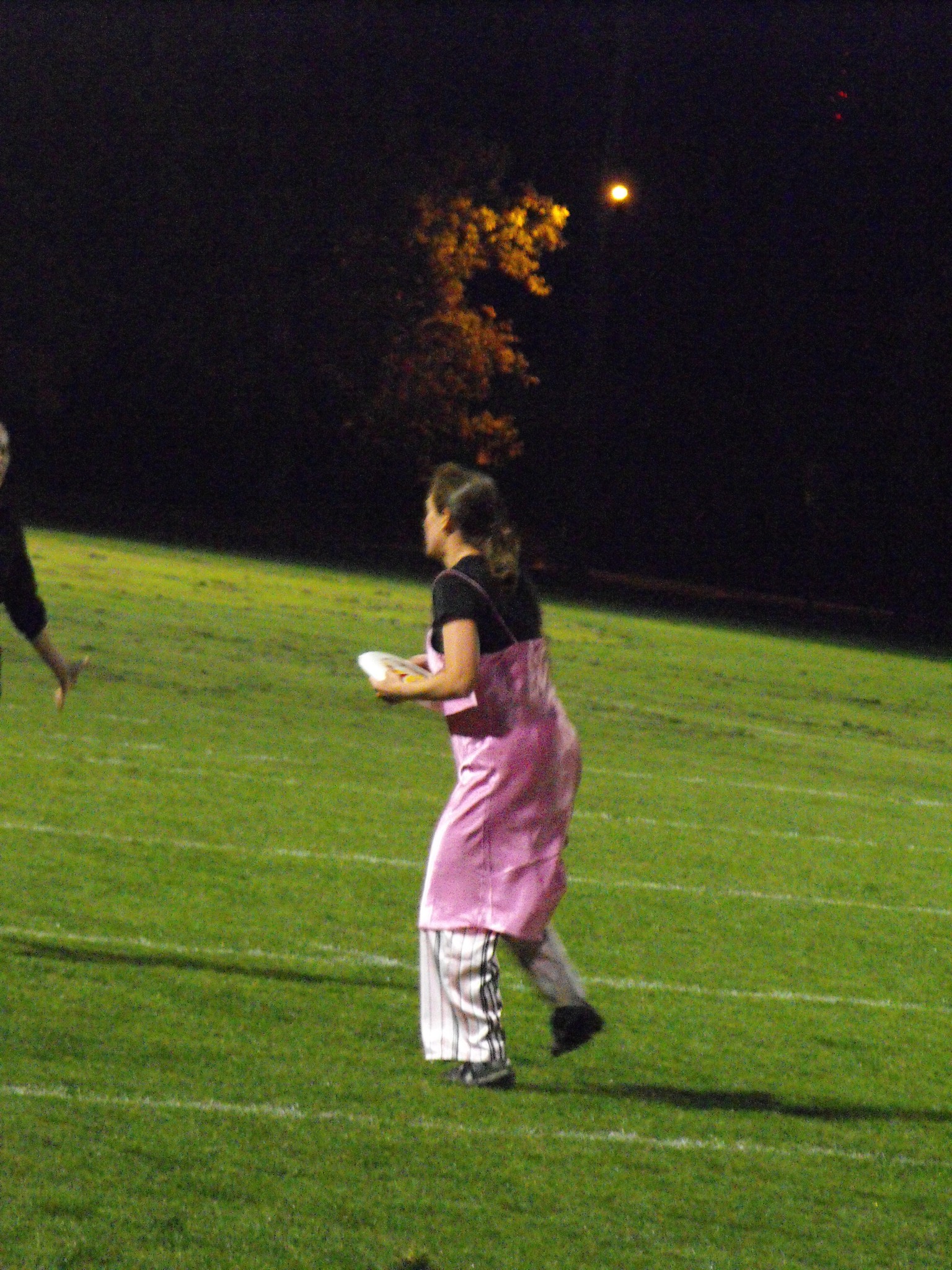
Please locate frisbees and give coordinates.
[358,649,437,708]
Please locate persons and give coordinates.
[368,461,605,1088]
[0,423,90,714]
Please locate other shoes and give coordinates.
[550,1000,604,1058]
[440,1060,514,1090]
